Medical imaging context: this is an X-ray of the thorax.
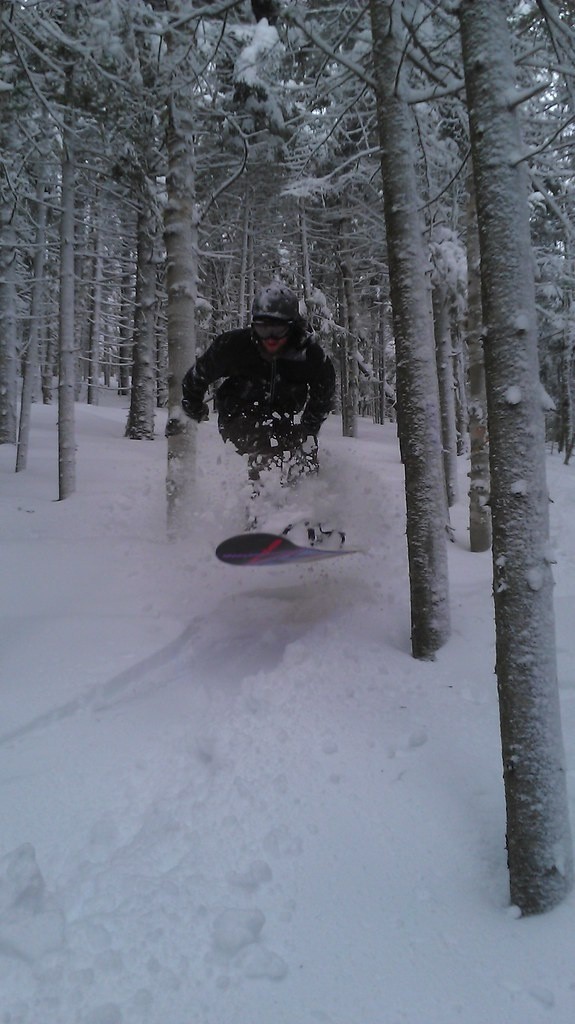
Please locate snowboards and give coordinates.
[214,532,356,565]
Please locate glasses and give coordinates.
[256,319,293,340]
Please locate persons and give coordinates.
[182,285,338,530]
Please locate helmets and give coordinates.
[252,286,299,320]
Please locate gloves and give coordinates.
[182,399,209,418]
[295,421,321,436]
